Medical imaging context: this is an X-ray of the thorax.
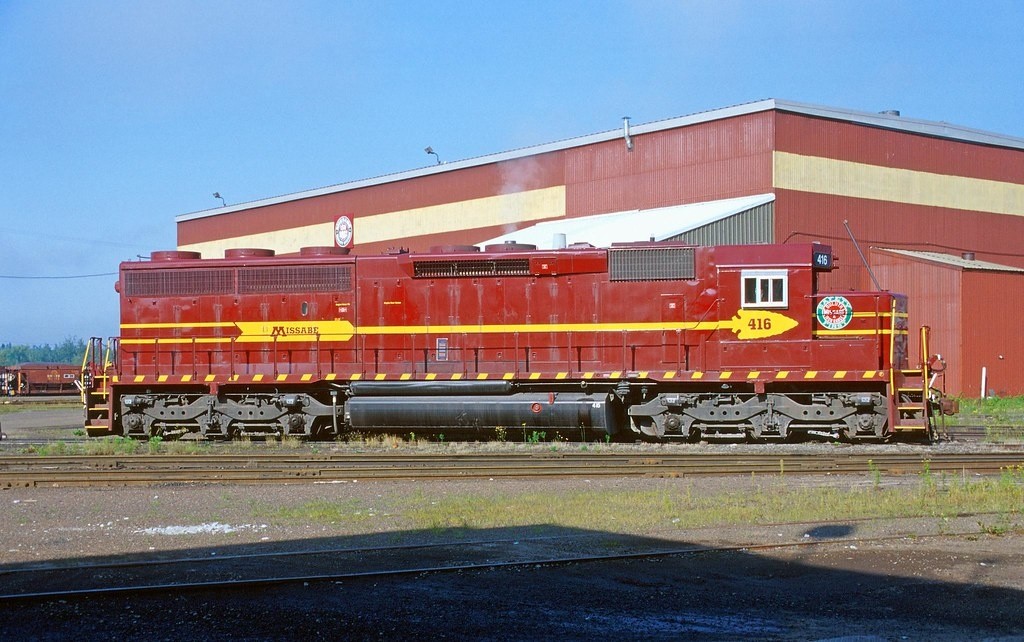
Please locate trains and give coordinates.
[79,221,959,444]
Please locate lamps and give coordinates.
[212,191,226,207]
[424,146,441,164]
